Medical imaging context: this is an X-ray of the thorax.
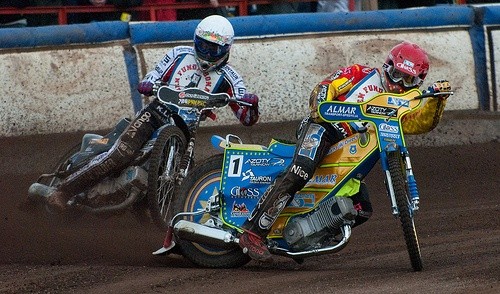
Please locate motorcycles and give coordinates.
[23,84,253,228]
[150,85,456,273]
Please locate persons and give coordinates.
[238,42,451,263]
[46,15,259,215]
[89,0,364,12]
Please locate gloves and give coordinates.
[432,79,451,100]
[239,93,259,110]
[310,106,326,123]
[137,81,154,98]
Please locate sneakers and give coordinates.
[238,231,274,264]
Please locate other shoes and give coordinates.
[46,181,69,214]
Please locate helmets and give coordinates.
[191,14,235,73]
[382,40,430,93]
